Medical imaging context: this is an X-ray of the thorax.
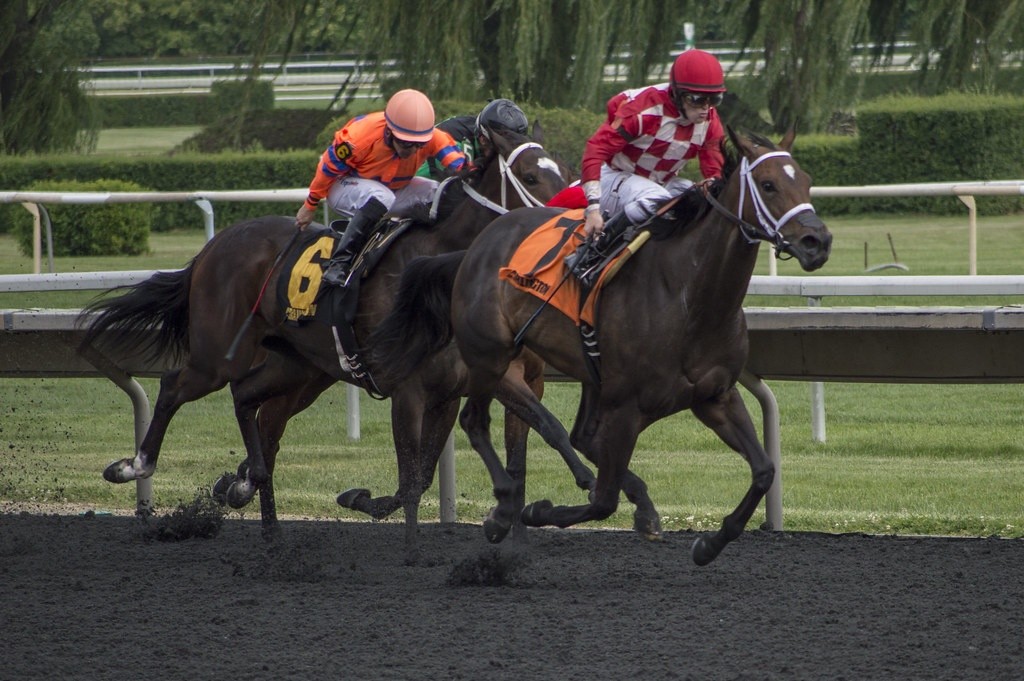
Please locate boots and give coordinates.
[320,195,388,289]
[562,205,636,289]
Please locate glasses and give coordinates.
[677,90,723,109]
[392,135,426,149]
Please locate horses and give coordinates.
[74,115,832,565]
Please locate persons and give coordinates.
[564,49,727,288]
[294,89,466,287]
[414,98,529,182]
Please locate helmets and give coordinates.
[475,99,528,151]
[669,49,727,90]
[384,89,435,142]
